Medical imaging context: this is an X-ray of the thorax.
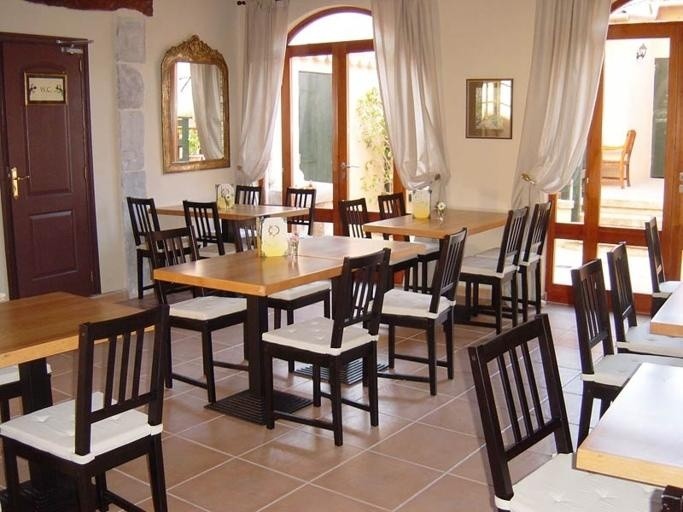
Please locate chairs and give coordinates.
[601,130,637,189]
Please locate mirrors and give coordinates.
[161,35,230,175]
[465,79,513,140]
